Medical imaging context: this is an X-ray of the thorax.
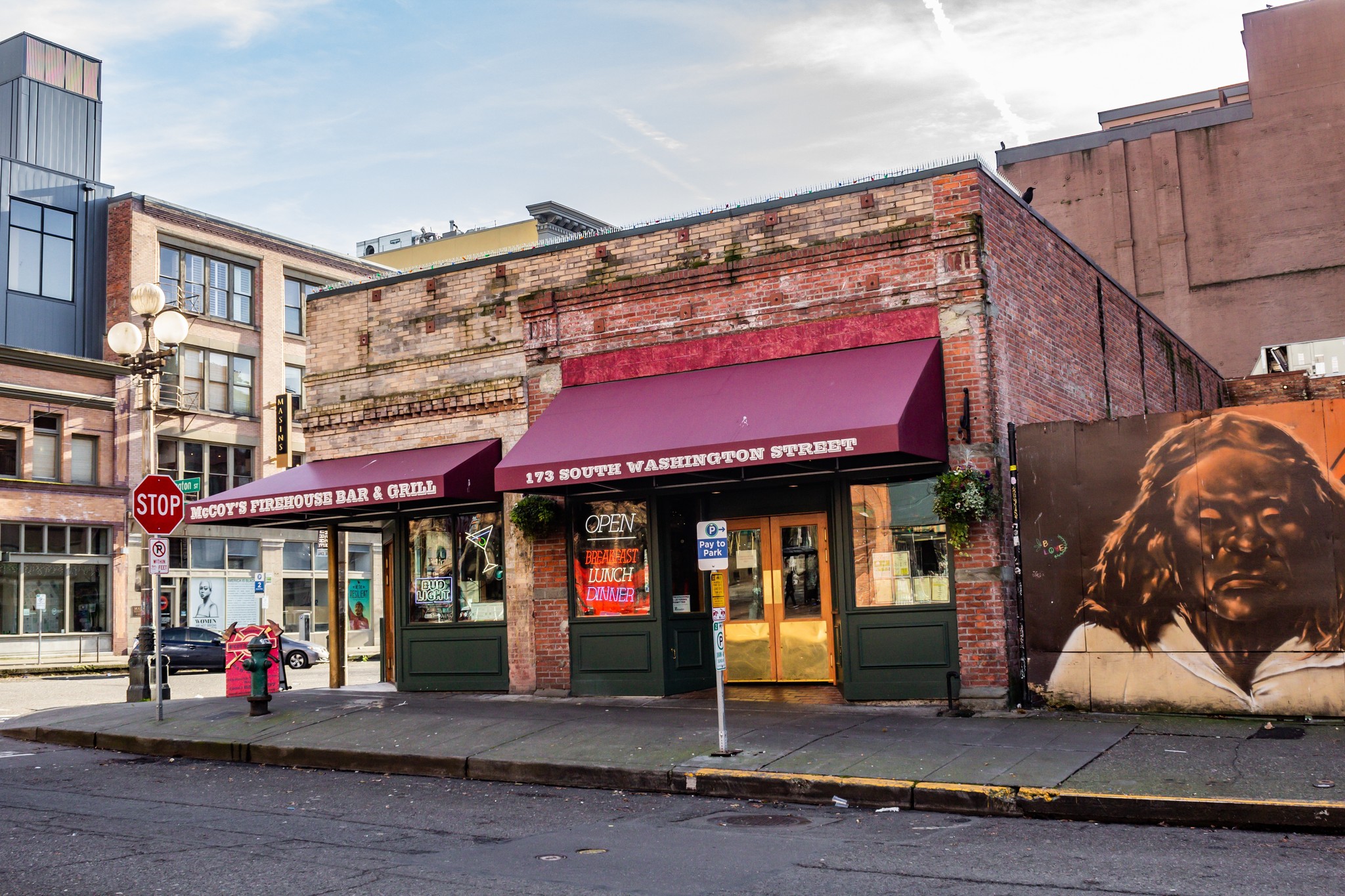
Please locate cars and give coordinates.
[128,625,226,675]
[280,635,330,669]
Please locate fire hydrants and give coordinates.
[241,632,274,716]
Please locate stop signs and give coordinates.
[132,475,185,536]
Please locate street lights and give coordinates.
[107,282,190,704]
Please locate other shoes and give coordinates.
[785,606,789,609]
[815,600,821,604]
[793,604,800,607]
[804,600,809,605]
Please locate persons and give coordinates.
[785,571,800,608]
[348,579,369,630]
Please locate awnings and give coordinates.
[185,438,502,531]
[494,336,948,498]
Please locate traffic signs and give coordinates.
[696,520,729,571]
